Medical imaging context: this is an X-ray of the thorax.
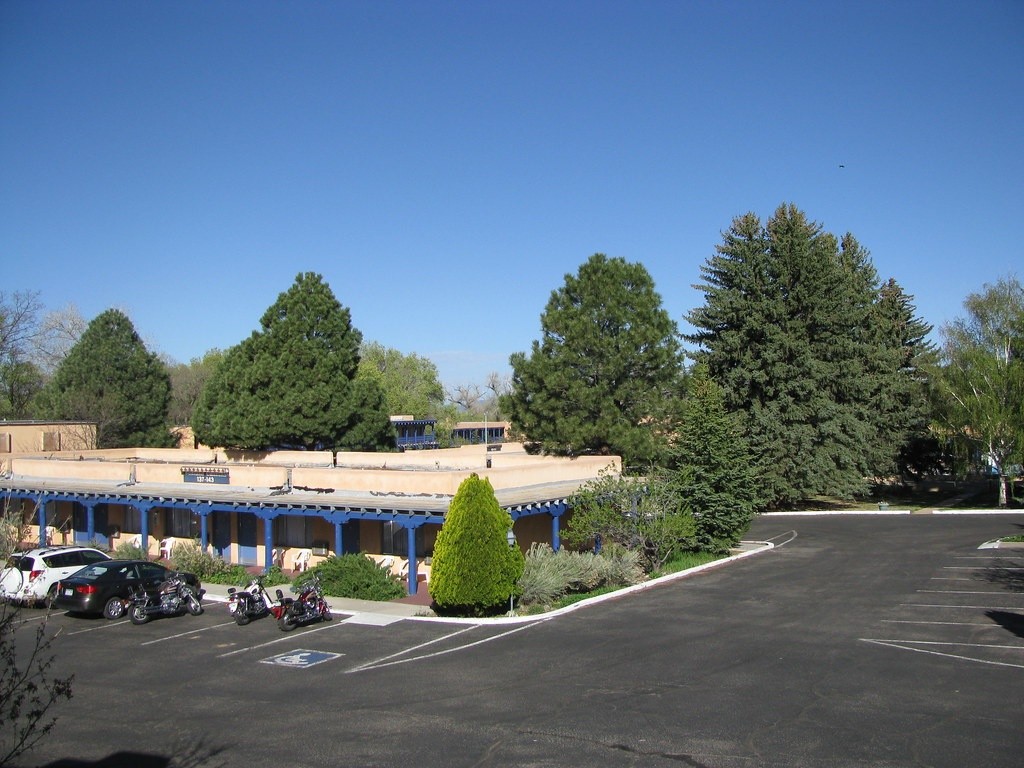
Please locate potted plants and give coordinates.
[878,502,889,510]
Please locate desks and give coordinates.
[416,571,430,585]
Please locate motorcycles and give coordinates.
[226,575,273,626]
[269,572,333,631]
[125,573,201,625]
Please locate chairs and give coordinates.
[291,549,312,573]
[124,570,135,579]
[158,537,176,560]
[38,527,54,545]
[380,556,394,579]
[399,559,420,582]
[271,548,286,568]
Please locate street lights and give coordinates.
[484,411,489,444]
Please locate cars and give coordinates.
[0,545,115,609]
[55,560,201,620]
